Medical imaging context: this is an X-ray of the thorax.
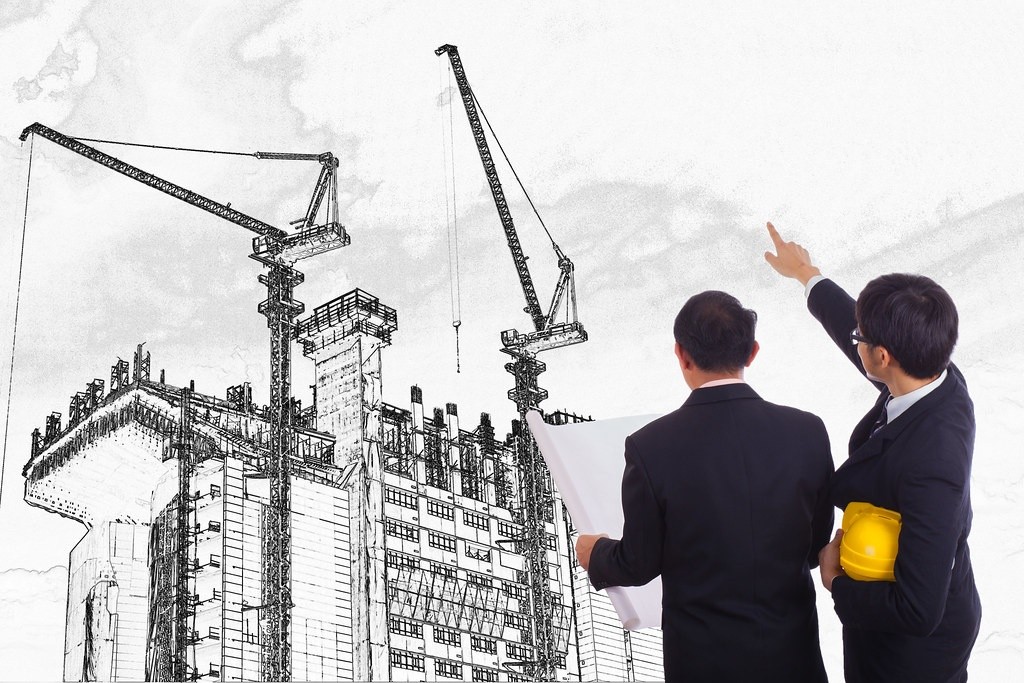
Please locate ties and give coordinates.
[869,396,893,439]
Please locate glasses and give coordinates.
[849,327,871,346]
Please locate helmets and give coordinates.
[838,502,902,582]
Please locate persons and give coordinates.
[574,289,837,682]
[764,220,984,683]
